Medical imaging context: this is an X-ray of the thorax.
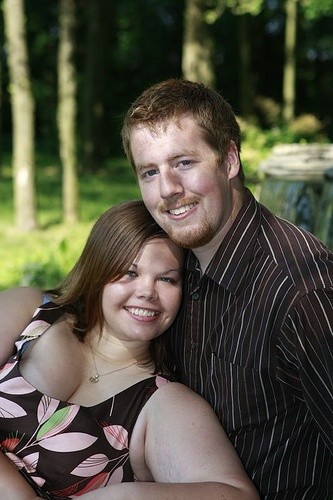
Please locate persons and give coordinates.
[119,77,333,498]
[1,195,257,500]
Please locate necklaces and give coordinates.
[82,335,152,384]
